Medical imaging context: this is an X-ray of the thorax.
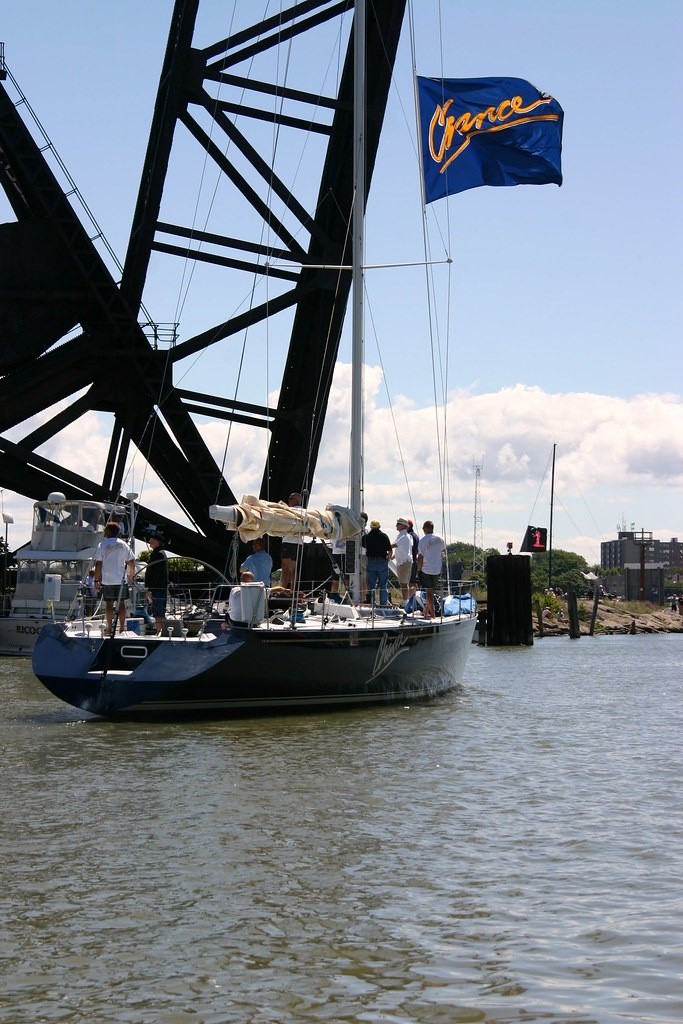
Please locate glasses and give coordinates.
[289,496,295,501]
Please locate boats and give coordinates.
[0,0,483,717]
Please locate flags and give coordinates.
[415,76,563,204]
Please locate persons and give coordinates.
[391,518,445,619]
[85,570,98,597]
[280,493,302,592]
[541,584,683,619]
[145,533,169,635]
[362,522,391,605]
[93,522,136,635]
[222,572,266,631]
[240,538,273,587]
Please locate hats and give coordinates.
[397,518,410,528]
[370,519,379,528]
[408,519,413,527]
[149,534,165,542]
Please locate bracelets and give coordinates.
[94,580,99,583]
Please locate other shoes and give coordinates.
[103,629,112,636]
